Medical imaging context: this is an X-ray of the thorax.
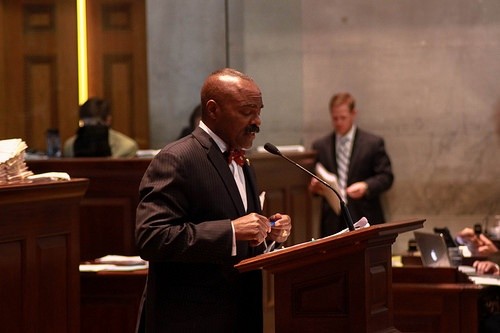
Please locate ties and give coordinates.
[335,138,350,204]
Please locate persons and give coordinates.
[64,98,139,158]
[458,227,500,278]
[308,92,394,239]
[135,69,291,333]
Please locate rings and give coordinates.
[282,229,287,237]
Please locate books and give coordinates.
[315,163,347,214]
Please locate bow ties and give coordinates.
[225,141,246,167]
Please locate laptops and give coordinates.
[413,230,500,278]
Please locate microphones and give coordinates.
[263,143,355,232]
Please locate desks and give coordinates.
[391,266,500,333]
